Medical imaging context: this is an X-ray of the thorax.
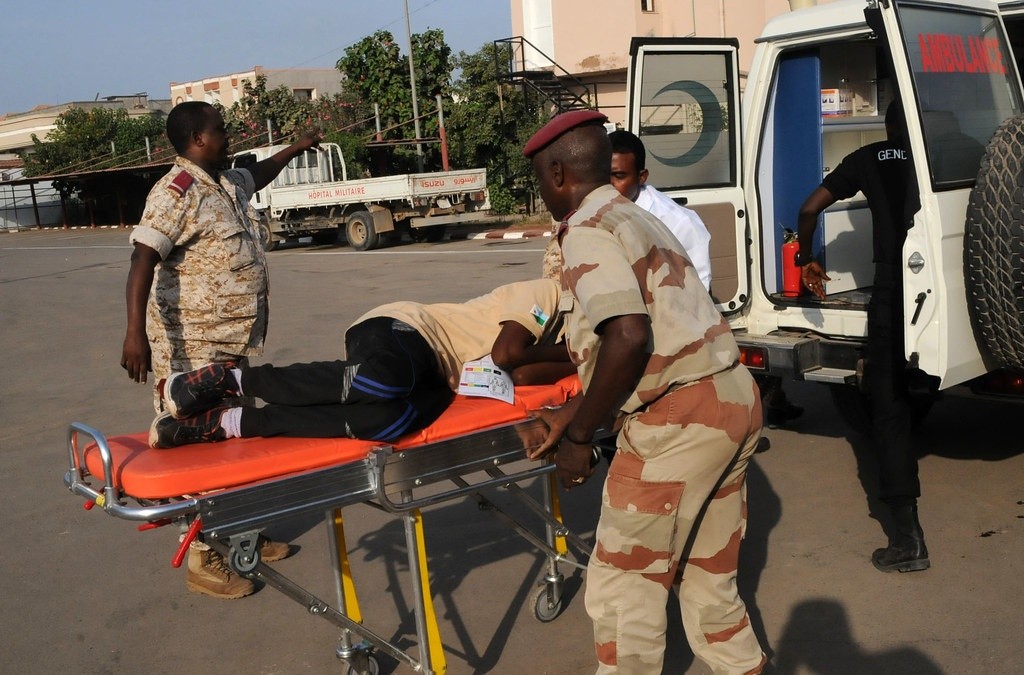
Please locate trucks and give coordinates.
[228,141,490,250]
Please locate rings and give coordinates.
[809,284,812,286]
[564,488,571,492]
[572,477,584,483]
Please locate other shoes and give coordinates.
[767,406,804,428]
[753,437,770,454]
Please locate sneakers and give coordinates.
[164,362,249,419]
[149,399,238,449]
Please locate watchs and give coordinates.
[794,250,815,265]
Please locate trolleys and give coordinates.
[60,372,620,675]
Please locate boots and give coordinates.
[873,505,930,573]
[218,537,291,562]
[179,533,255,599]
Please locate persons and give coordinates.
[149,275,577,443]
[120,101,327,599]
[523,110,774,675]
[792,93,1003,575]
[602,128,714,466]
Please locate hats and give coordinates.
[524,110,608,159]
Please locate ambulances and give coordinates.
[623,0,1024,404]
